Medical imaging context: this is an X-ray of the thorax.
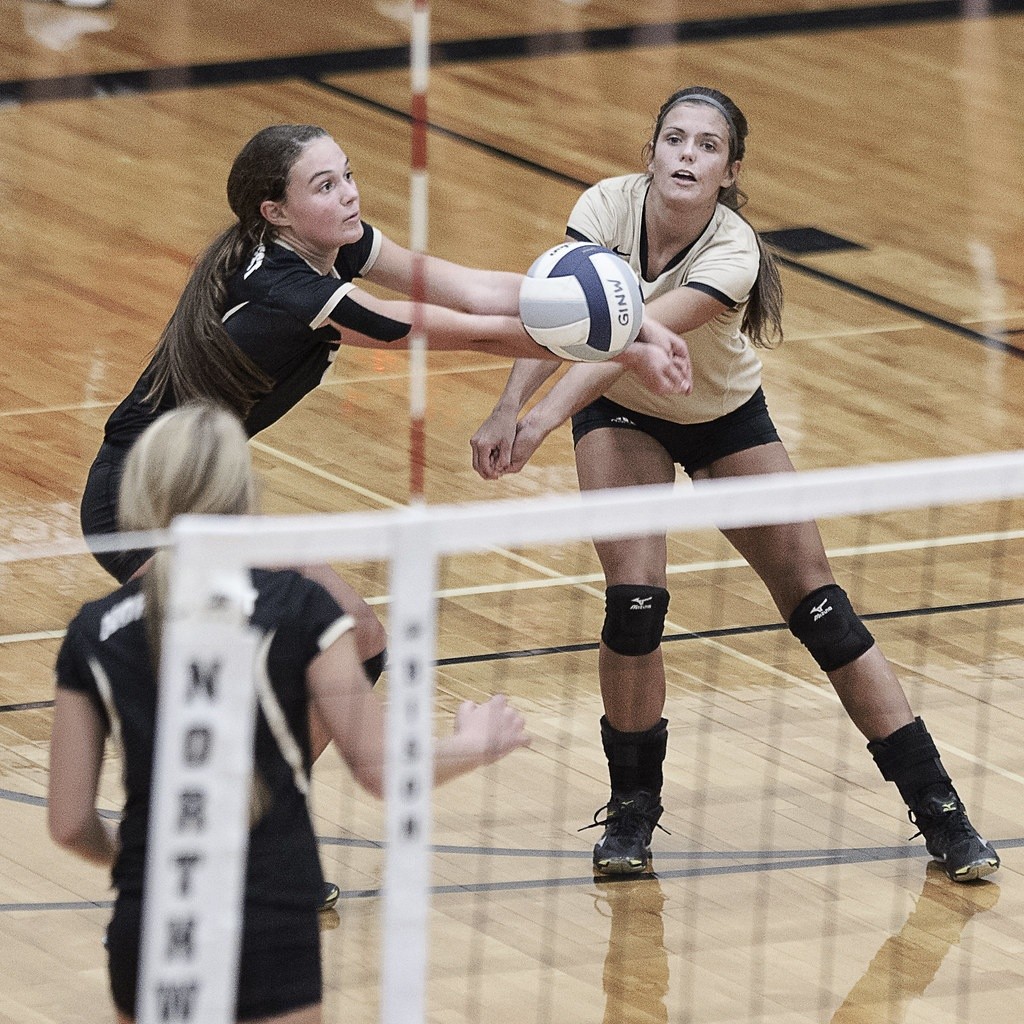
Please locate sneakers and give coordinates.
[906,781,1002,882]
[578,775,672,874]
[317,882,338,913]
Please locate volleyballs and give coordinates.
[518,241,642,364]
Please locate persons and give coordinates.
[468,86,1001,886]
[38,396,531,1024]
[80,128,692,913]
